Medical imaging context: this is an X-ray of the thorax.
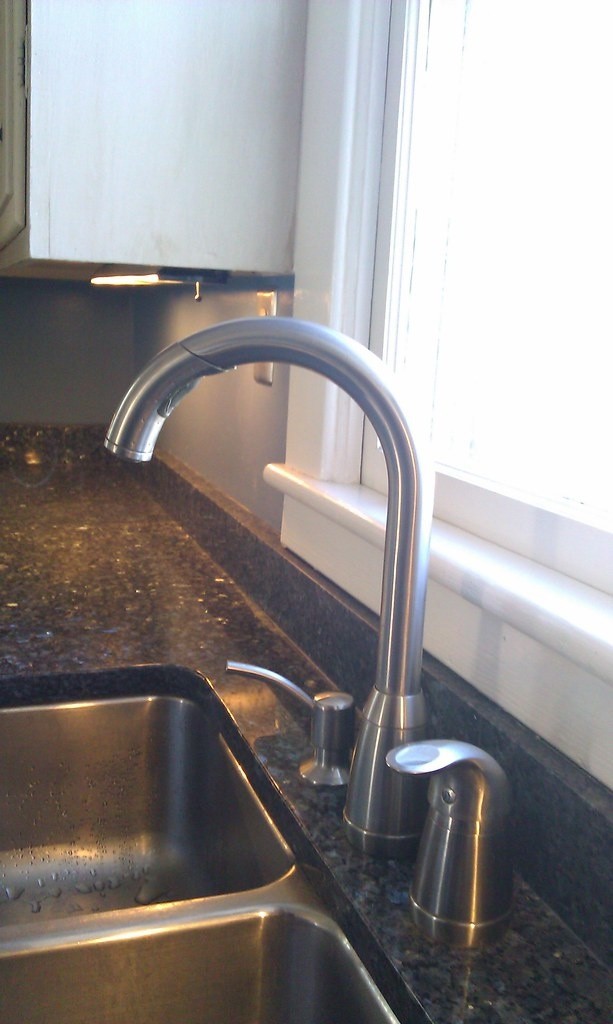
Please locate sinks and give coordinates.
[1,910,406,1024]
[1,690,304,923]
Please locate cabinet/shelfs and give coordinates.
[0,0,310,282]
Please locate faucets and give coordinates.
[97,316,443,858]
